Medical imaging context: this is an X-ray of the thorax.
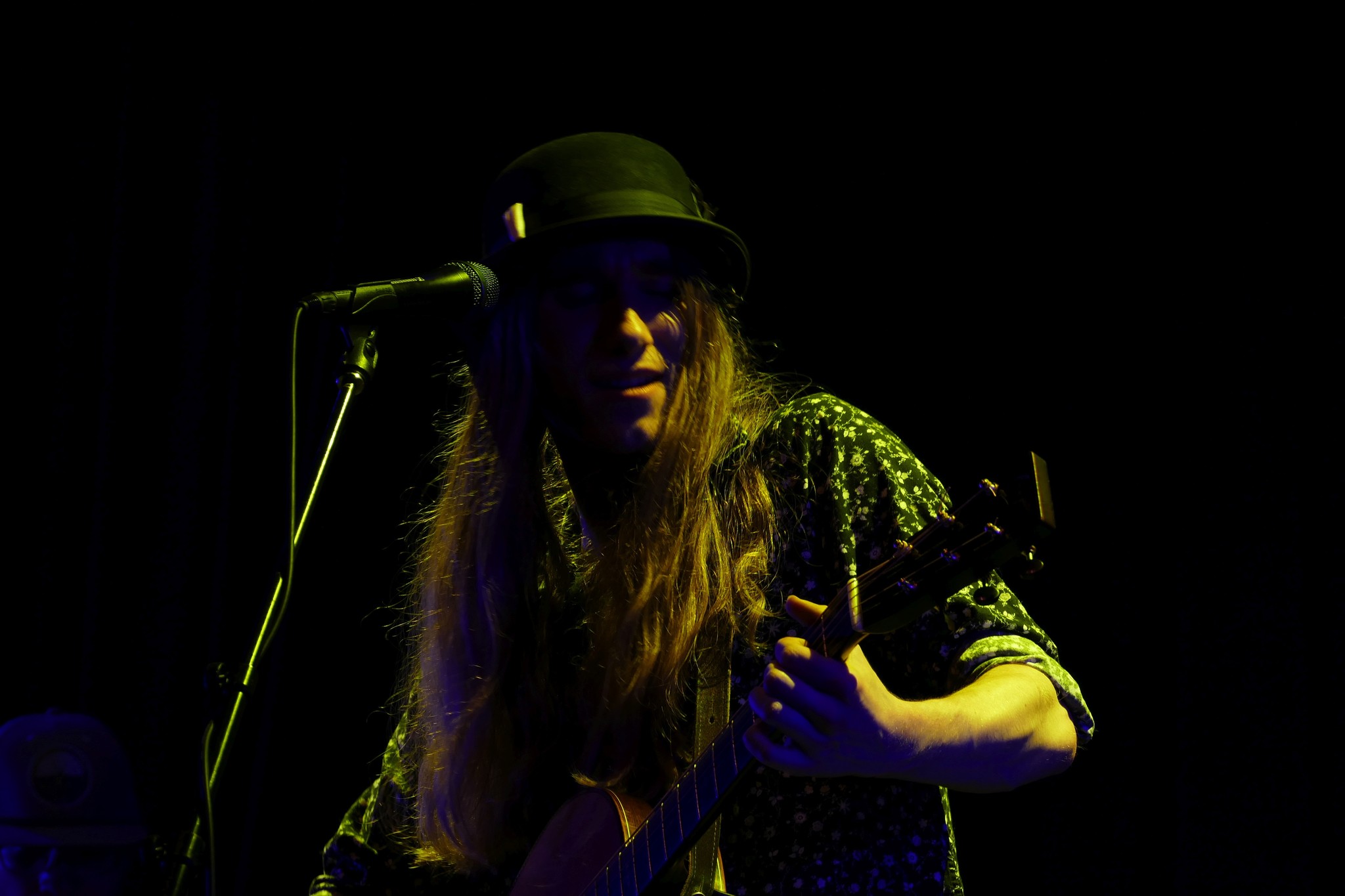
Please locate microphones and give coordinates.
[299,261,501,317]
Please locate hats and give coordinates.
[483,132,754,306]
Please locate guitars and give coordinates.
[510,452,1057,896]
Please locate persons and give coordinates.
[309,130,1093,896]
